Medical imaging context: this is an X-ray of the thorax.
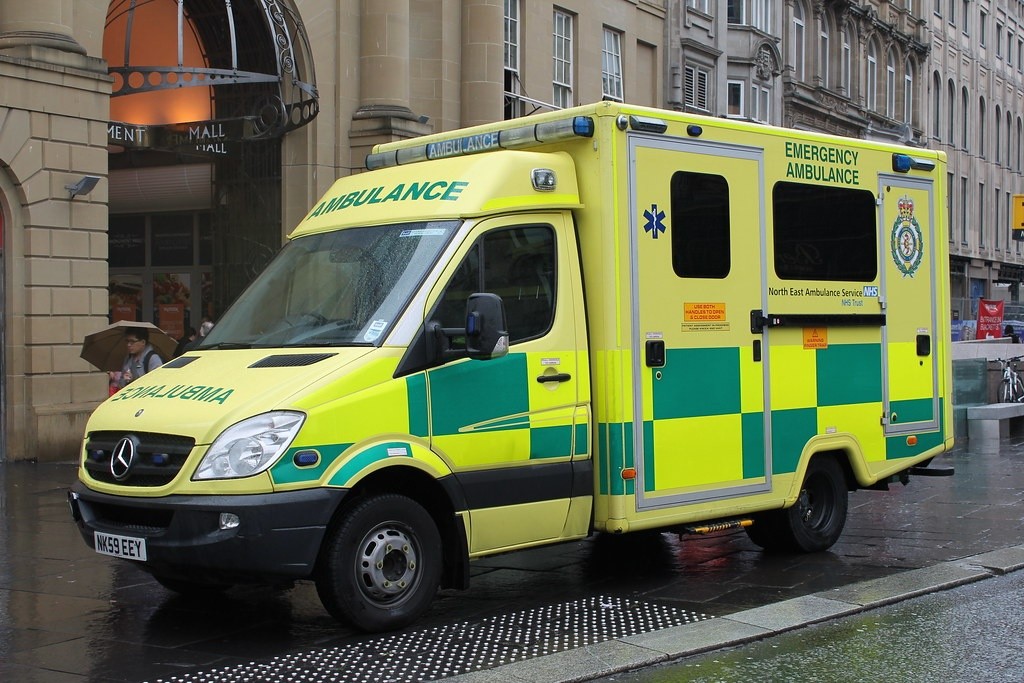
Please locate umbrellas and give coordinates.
[78,319,181,373]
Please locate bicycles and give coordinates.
[994,355,1024,405]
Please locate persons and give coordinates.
[118,326,165,391]
[1002,324,1023,344]
[169,317,215,360]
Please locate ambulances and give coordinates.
[66,98,957,637]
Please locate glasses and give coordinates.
[125,339,140,345]
